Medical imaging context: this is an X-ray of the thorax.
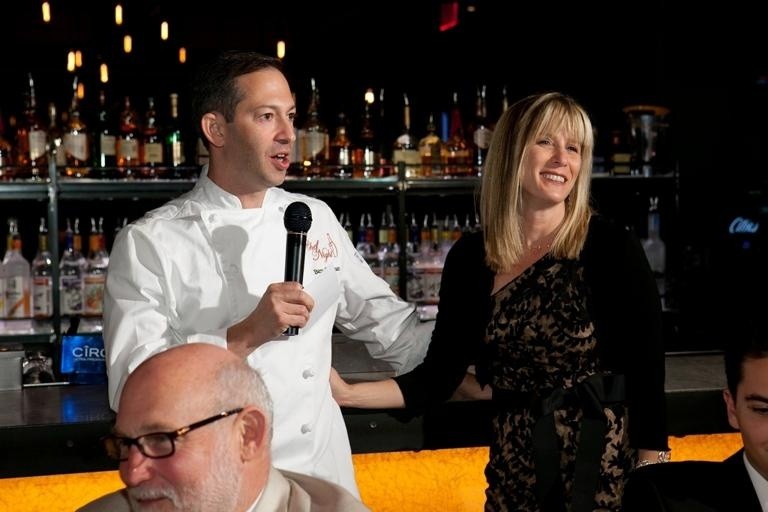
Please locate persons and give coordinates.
[102,56,496,503]
[329,92,673,512]
[68,343,371,512]
[623,313,768,512]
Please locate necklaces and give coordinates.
[514,231,558,254]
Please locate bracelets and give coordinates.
[636,445,672,466]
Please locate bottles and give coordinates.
[0,217,130,317]
[338,209,480,305]
[0,73,513,178]
[643,208,666,311]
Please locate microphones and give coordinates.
[283,202,312,336]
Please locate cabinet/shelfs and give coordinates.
[0,160,676,393]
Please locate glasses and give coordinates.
[105,408,243,461]
